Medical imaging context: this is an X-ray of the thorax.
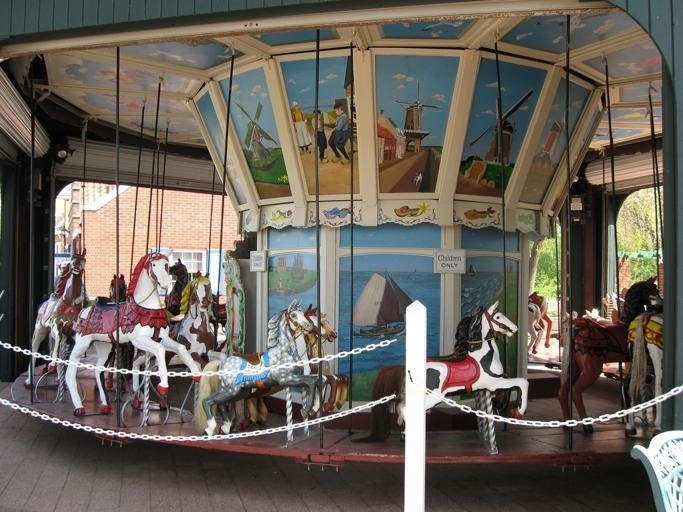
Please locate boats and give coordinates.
[466,263,477,276]
[352,272,413,337]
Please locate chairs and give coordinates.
[629,429,683,512]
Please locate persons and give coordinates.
[327,102,350,165]
[311,109,328,163]
[291,100,311,153]
